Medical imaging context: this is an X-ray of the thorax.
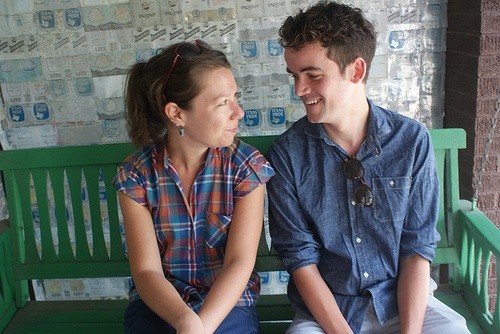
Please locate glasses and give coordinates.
[342,158,375,207]
[161,39,217,96]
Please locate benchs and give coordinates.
[0,127,500,334]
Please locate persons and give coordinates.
[265,1,473,334]
[109,41,276,334]
